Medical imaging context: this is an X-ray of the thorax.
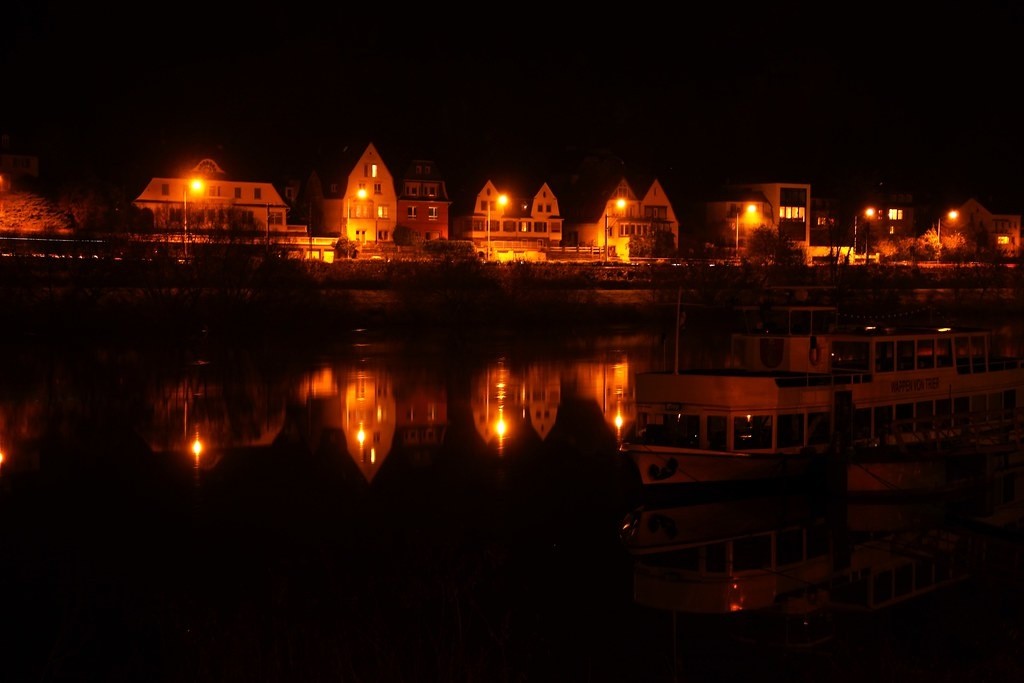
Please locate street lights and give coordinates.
[183,175,211,259]
[355,187,379,244]
[735,203,760,254]
[604,197,628,262]
[936,208,960,264]
[486,192,511,260]
[863,207,876,265]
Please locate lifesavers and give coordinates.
[808,344,822,366]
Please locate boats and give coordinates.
[611,454,1024,650]
[608,279,1024,490]
